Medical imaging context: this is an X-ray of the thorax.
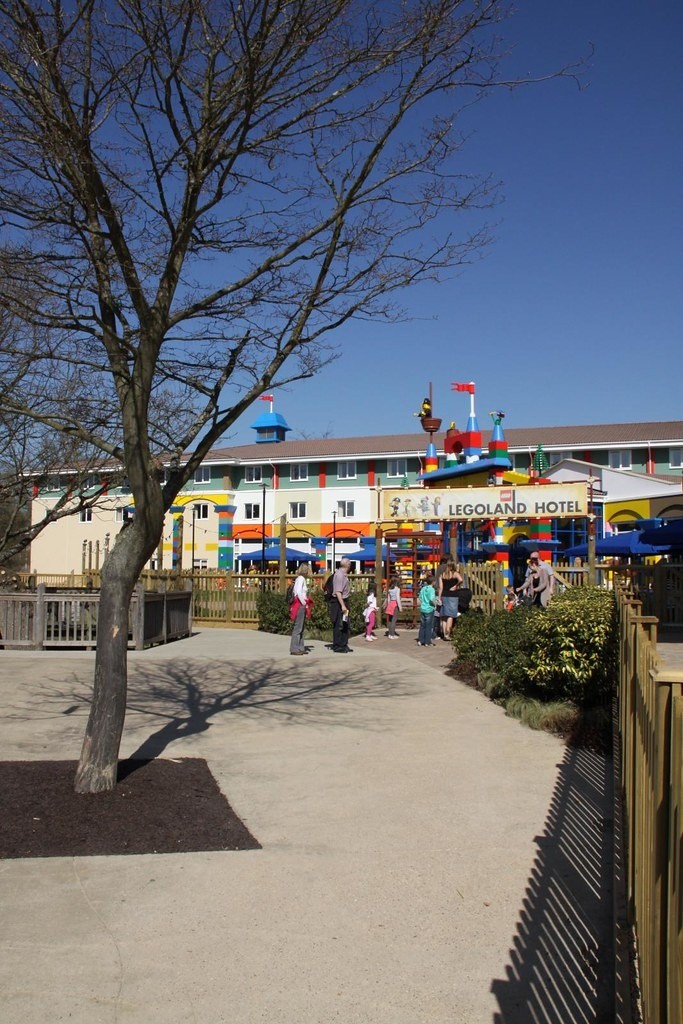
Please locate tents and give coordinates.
[236,545,319,569]
[566,529,675,565]
[342,546,397,565]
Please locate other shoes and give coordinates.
[389,634,398,639]
[425,642,436,646]
[291,651,303,655]
[365,635,373,642]
[301,650,308,654]
[344,646,353,652]
[335,648,348,652]
[443,635,453,640]
[431,636,440,641]
[370,635,377,640]
[417,641,422,646]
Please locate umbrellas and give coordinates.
[637,521,683,557]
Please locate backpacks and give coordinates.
[324,571,337,602]
[286,582,295,604]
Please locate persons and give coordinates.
[503,585,518,611]
[573,557,584,585]
[514,552,556,609]
[388,576,402,640]
[418,575,437,647]
[289,564,309,655]
[438,560,462,641]
[331,558,353,653]
[365,582,378,642]
[414,398,432,418]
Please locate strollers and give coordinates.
[513,590,538,609]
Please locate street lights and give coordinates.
[191,508,198,574]
[331,510,338,573]
[259,483,270,593]
